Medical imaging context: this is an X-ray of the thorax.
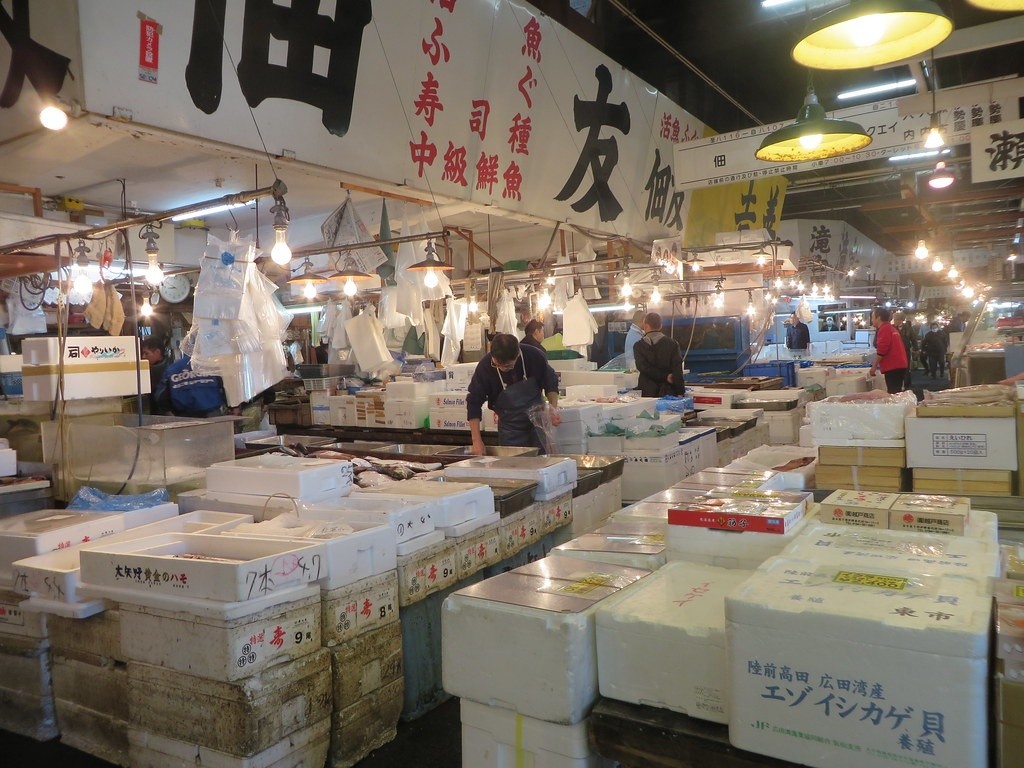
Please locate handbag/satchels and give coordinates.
[259,411,277,430]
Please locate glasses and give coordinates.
[490,360,513,367]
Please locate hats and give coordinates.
[893,312,906,321]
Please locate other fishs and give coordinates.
[278,444,443,488]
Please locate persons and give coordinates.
[820,305,971,394]
[784,311,810,358]
[625,311,686,396]
[139,338,275,434]
[466,319,561,455]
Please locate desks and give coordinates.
[965,350,1004,387]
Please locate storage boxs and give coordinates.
[0,209,117,261]
[118,218,176,264]
[0,310,1024,768]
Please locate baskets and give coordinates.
[302,375,344,391]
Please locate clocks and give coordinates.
[159,273,191,304]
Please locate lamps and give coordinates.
[286,254,328,301]
[172,199,257,221]
[754,2,874,160]
[406,238,456,292]
[790,0,955,71]
[331,252,374,298]
[925,47,945,149]
[927,153,957,189]
